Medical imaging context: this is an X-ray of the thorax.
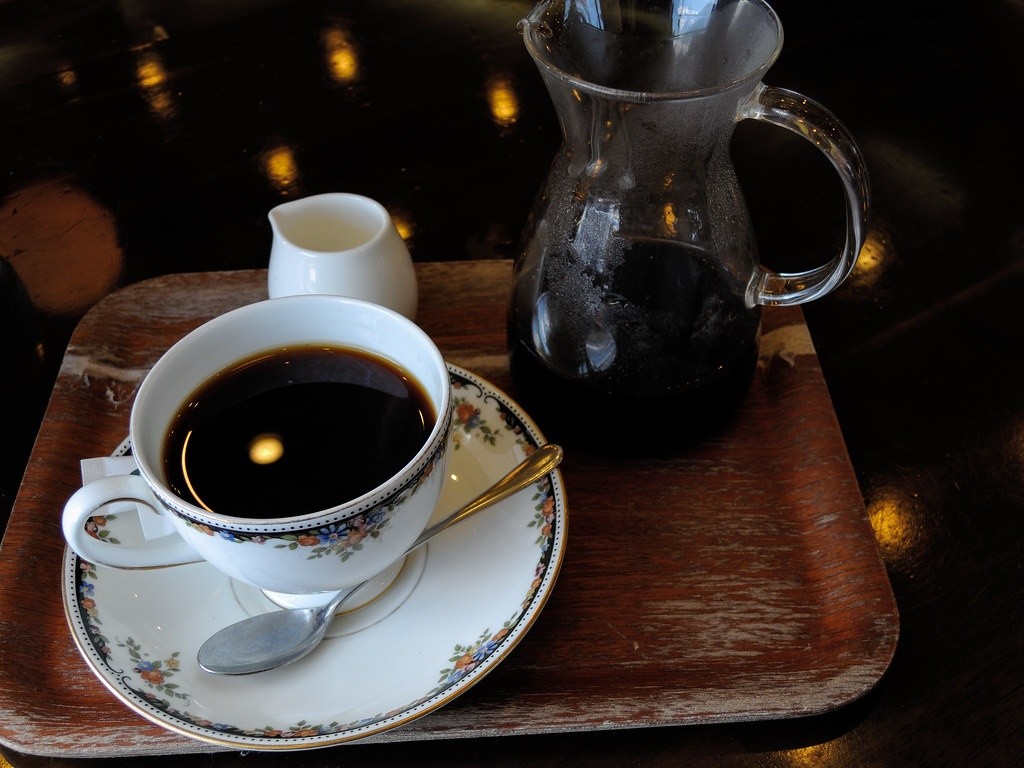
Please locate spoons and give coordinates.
[197,445,563,675]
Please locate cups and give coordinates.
[62,298,451,591]
[268,193,418,321]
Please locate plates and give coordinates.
[61,361,565,751]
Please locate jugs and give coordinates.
[512,1,872,453]
